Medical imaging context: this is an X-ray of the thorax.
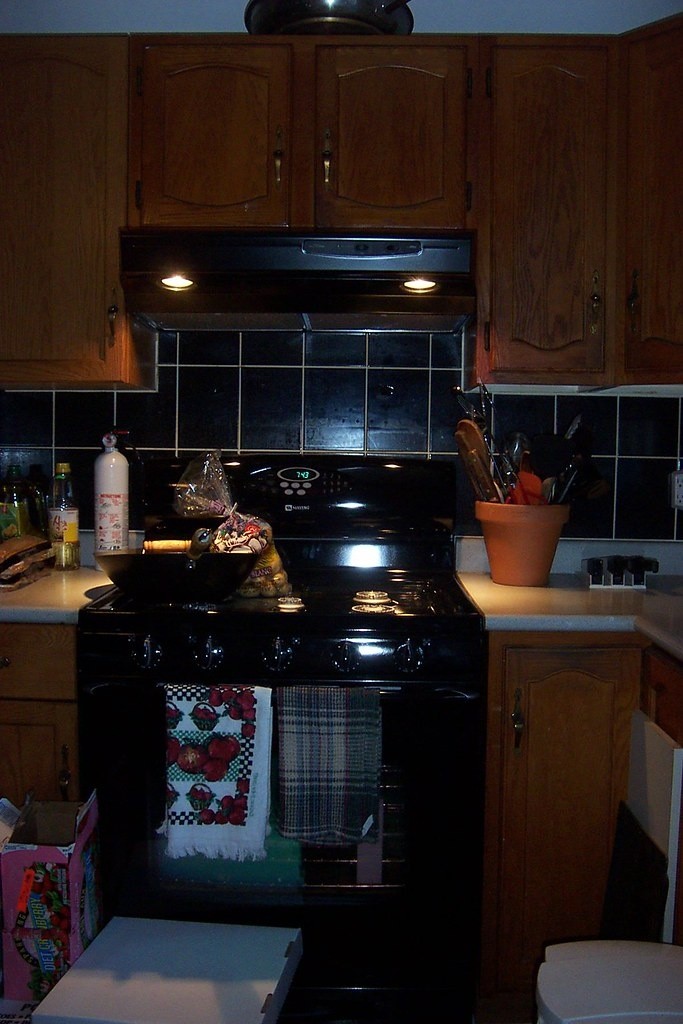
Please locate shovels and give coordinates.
[457,419,488,469]
[512,471,542,506]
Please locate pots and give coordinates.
[94,528,258,608]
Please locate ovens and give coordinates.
[78,683,486,993]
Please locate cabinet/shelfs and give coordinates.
[0,34,683,389]
[474,630,642,987]
[0,619,80,806]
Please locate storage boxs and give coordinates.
[0,789,106,1005]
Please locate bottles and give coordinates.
[0,465,29,543]
[48,462,81,571]
[93,434,129,570]
[28,464,52,535]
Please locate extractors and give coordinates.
[119,229,476,331]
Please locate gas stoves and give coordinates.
[81,458,485,680]
